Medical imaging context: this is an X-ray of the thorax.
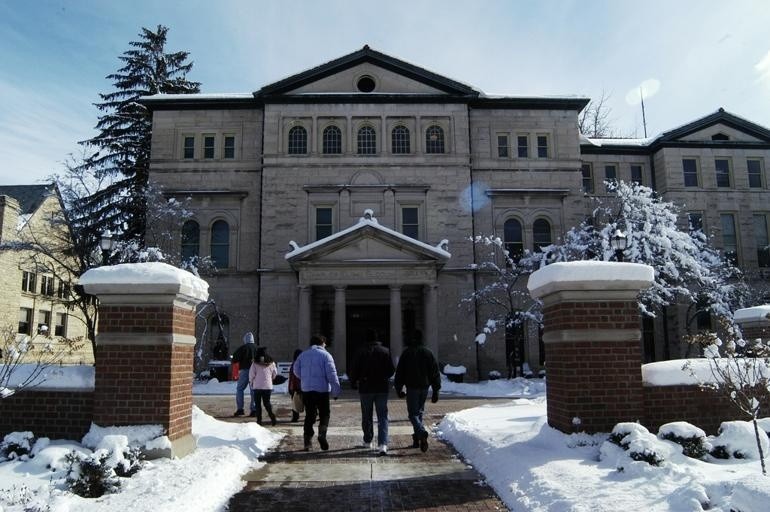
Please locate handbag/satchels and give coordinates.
[292,391,303,413]
[232,362,239,380]
[273,375,288,384]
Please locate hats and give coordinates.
[256,347,266,356]
[244,332,255,344]
[410,328,423,346]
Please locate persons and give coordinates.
[230,331,259,417]
[394,328,441,453]
[292,332,341,452]
[288,349,320,422]
[348,327,396,456]
[248,346,278,426]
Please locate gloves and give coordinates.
[397,392,405,398]
[432,390,439,403]
[351,379,359,390]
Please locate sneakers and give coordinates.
[234,409,244,415]
[250,410,256,417]
[257,417,276,426]
[362,436,388,456]
[413,432,428,452]
[306,434,328,451]
